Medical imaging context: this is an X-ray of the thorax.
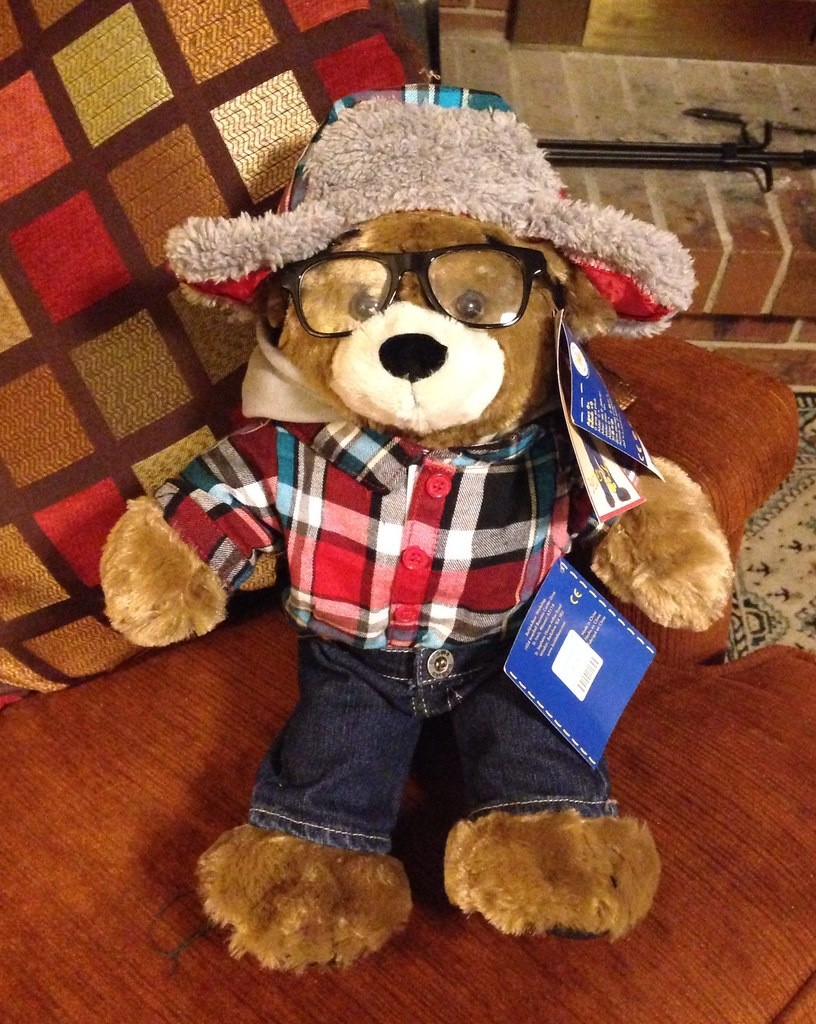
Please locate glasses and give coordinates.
[278,243,567,335]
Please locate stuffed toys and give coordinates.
[97,85,735,970]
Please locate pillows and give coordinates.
[3,0,415,702]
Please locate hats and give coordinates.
[165,86,697,335]
